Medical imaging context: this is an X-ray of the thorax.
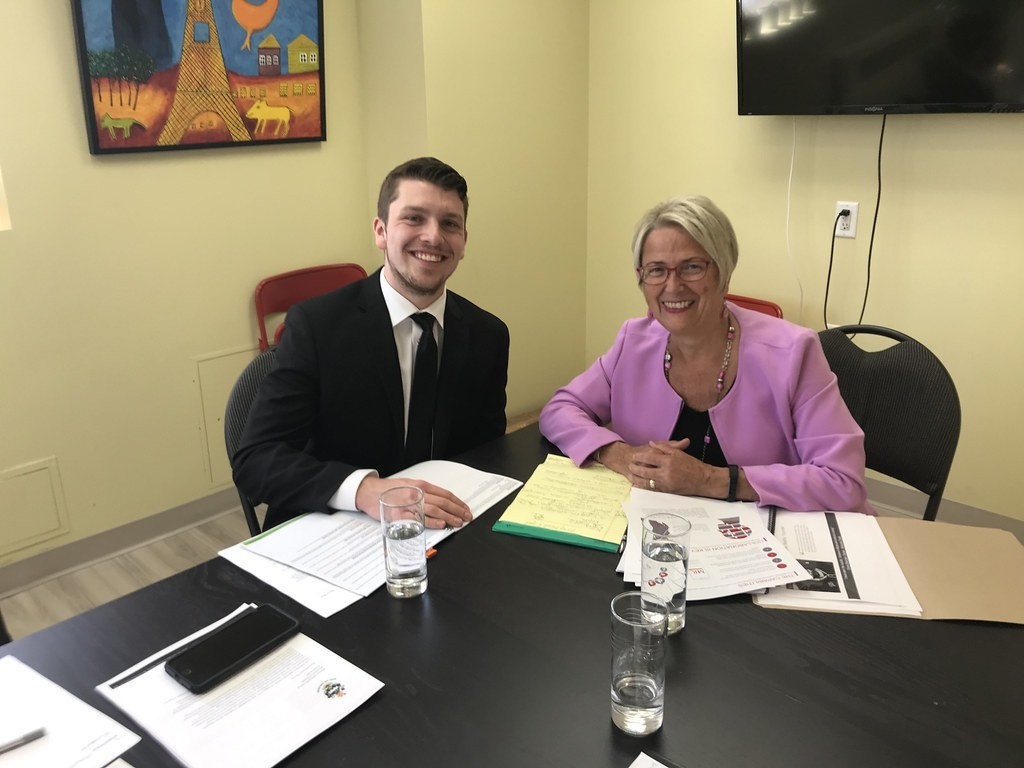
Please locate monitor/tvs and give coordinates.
[735,0,1024,116]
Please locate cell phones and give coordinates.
[165,603,302,695]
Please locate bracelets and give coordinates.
[727,465,738,502]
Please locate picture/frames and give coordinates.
[69,0,326,154]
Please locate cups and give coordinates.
[378,487,428,600]
[640,513,692,637]
[608,589,669,738]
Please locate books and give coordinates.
[617,487,922,619]
[239,461,525,596]
[0,655,143,768]
[492,452,633,553]
[216,512,437,618]
[101,756,138,768]
[94,601,386,768]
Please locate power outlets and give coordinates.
[834,201,859,239]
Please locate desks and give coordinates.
[1,421,1023,768]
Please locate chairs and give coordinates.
[727,294,782,318]
[224,346,277,538]
[815,325,961,522]
[255,263,368,350]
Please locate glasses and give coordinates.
[636,259,712,286]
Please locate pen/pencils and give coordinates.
[0,726,48,754]
[620,530,626,554]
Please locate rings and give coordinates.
[650,480,656,489]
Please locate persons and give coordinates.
[538,195,880,516]
[232,158,510,528]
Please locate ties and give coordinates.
[405,313,438,469]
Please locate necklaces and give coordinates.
[664,310,736,463]
